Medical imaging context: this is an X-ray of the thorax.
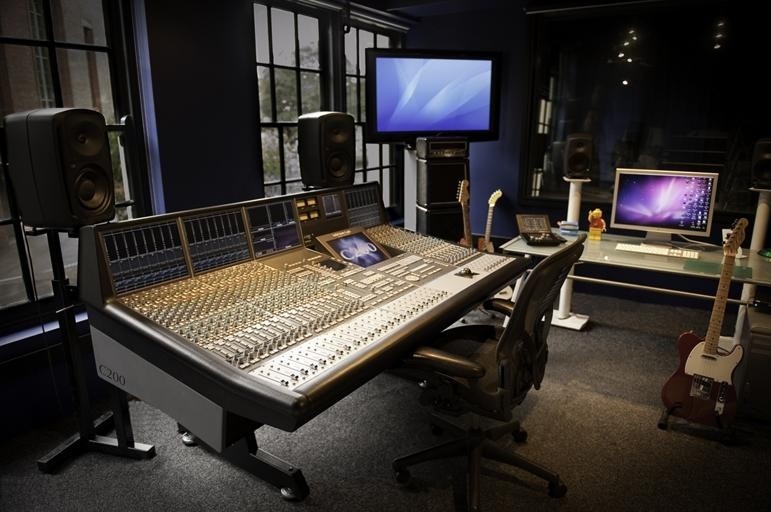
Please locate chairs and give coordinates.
[391,232,589,511]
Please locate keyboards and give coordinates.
[615,242,699,259]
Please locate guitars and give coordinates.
[661,218,749,431]
[456,179,513,301]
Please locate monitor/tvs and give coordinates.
[610,167,720,249]
[315,225,393,268]
[365,48,504,144]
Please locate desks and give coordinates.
[500,227,771,343]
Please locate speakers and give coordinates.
[3,107,115,228]
[298,111,355,187]
[751,137,771,189]
[563,133,593,179]
